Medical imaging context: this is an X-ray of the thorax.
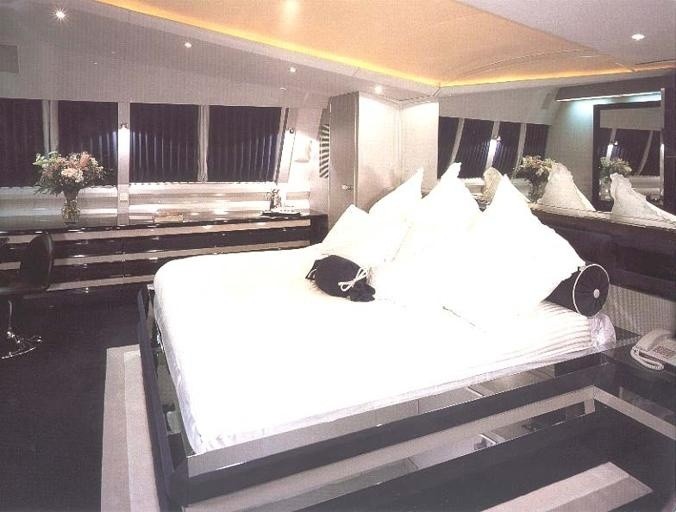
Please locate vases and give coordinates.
[61,199,80,224]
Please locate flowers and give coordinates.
[599,157,633,183]
[516,154,553,191]
[31,151,111,202]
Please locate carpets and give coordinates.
[100,341,676,512]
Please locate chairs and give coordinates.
[1,234,55,359]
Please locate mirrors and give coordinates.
[437,87,675,217]
[593,101,665,212]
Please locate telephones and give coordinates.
[632,329,676,367]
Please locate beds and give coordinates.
[137,240,640,512]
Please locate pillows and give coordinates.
[321,164,481,299]
[444,173,587,334]
[319,253,376,303]
[549,262,609,315]
